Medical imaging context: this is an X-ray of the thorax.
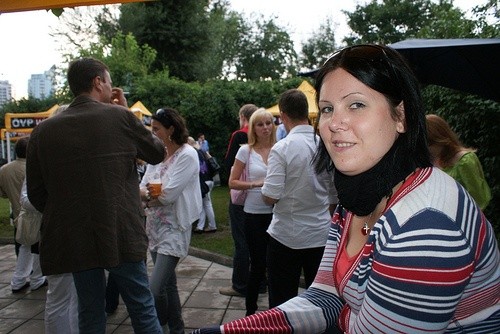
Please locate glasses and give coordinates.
[155,108,167,117]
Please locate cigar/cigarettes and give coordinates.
[112,92,130,94]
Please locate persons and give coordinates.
[0,107,220,334]
[26,58,167,334]
[217,97,255,299]
[421,107,495,218]
[231,110,285,315]
[193,42,500,333]
[261,89,338,309]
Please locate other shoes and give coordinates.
[204,227,217,232]
[12,281,31,293]
[218,288,243,296]
[193,228,203,233]
[31,276,48,290]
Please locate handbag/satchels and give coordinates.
[206,157,220,170]
[16,207,43,245]
[231,145,251,205]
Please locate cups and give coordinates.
[146,174,161,196]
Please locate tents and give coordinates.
[301,38,500,104]
[264,79,320,116]
[0,101,153,140]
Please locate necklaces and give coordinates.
[361,213,372,235]
